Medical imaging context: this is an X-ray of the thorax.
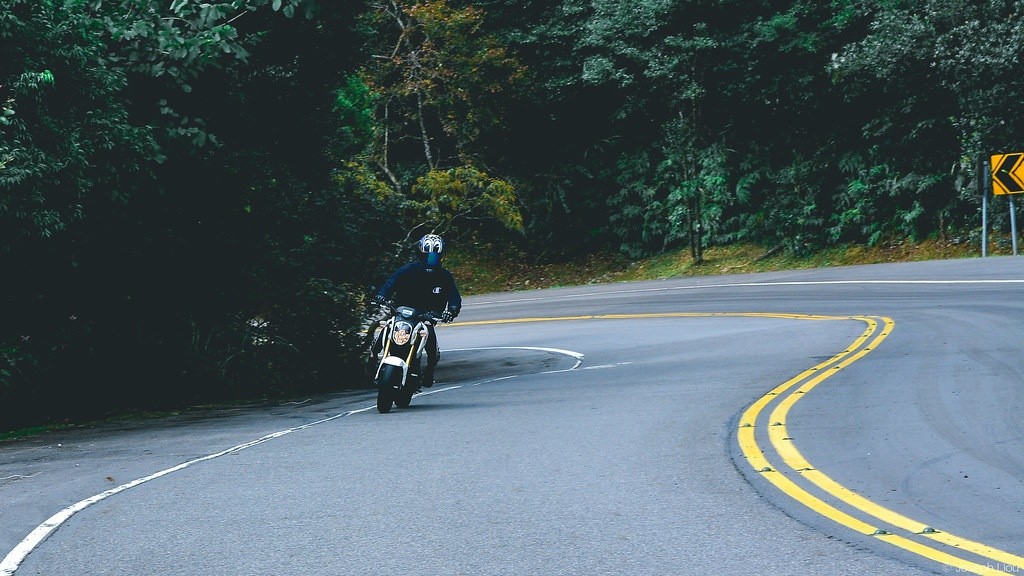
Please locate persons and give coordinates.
[364,234,462,388]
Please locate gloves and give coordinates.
[369,295,386,316]
[442,306,460,323]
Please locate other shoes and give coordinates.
[422,366,433,388]
[365,354,379,382]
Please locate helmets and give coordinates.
[417,234,446,270]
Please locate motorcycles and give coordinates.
[369,296,451,414]
